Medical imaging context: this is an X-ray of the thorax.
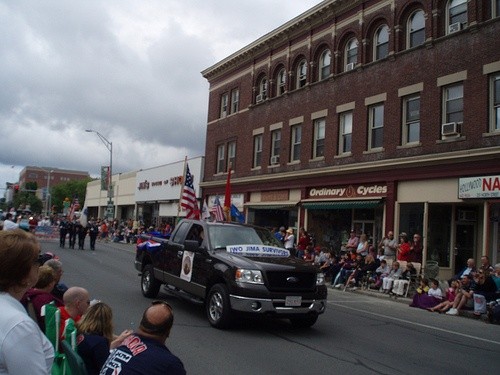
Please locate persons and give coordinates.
[58,286,91,365]
[272,226,500,324]
[26,266,63,334]
[35,247,68,302]
[0,196,180,250]
[97,301,187,375]
[0,228,55,375]
[77,302,132,375]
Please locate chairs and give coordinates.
[26,297,88,375]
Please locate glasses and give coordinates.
[152,300,172,310]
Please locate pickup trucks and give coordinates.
[135,218,326,328]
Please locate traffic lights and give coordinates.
[14,186,18,193]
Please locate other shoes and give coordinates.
[427,308,434,312]
[335,284,399,298]
[446,308,458,315]
[437,309,441,314]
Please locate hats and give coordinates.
[314,246,321,253]
[380,260,386,264]
[400,232,408,237]
[286,228,294,233]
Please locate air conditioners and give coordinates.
[346,62,355,71]
[271,156,280,164]
[441,121,461,136]
[448,22,463,34]
[256,94,263,103]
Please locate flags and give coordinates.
[224,166,231,222]
[180,160,199,220]
[232,205,243,221]
[212,194,225,222]
[200,198,211,219]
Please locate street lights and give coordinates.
[86,130,113,201]
